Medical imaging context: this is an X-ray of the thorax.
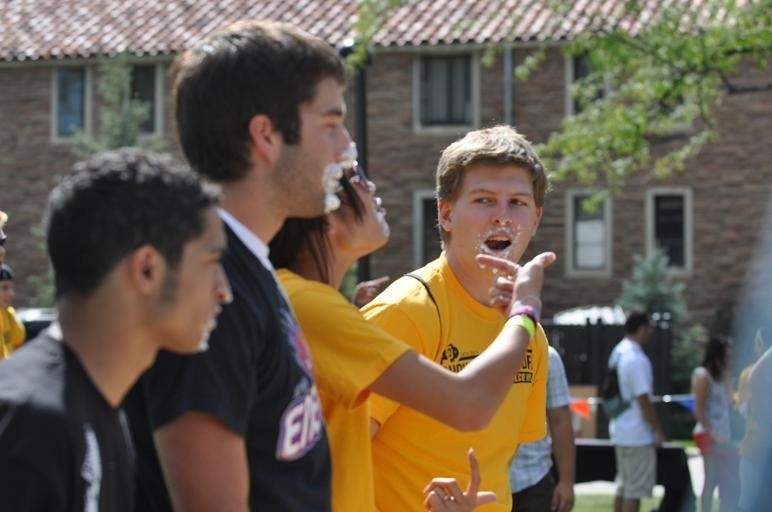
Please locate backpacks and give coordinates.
[596,353,633,423]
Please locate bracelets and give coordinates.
[502,293,544,342]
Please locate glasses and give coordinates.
[334,164,369,193]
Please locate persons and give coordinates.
[0,208,9,270]
[0,263,30,362]
[350,120,551,512]
[349,274,393,310]
[115,19,362,512]
[0,142,236,511]
[263,162,558,511]
[595,310,771,510]
[503,343,577,512]
